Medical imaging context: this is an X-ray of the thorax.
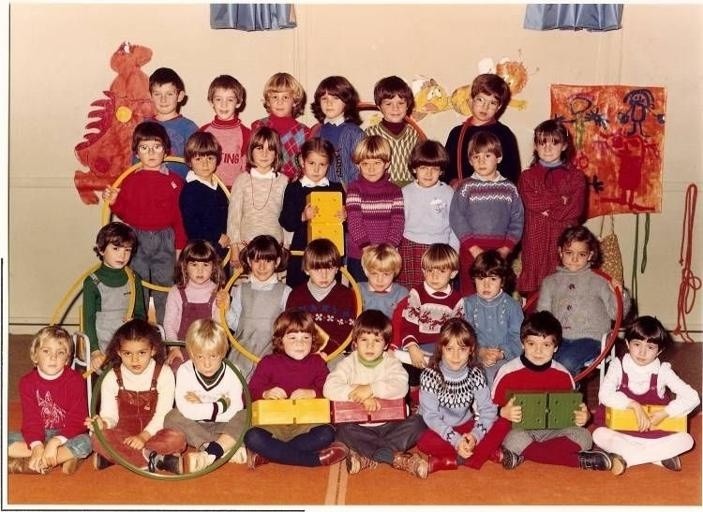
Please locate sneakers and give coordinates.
[156,453,183,475]
[577,450,626,476]
[62,458,79,475]
[8,458,41,475]
[490,446,524,470]
[187,451,216,473]
[227,444,248,464]
[94,452,115,471]
[662,456,682,471]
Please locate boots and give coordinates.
[428,455,458,474]
[345,449,378,474]
[392,452,429,480]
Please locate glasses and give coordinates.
[137,144,165,153]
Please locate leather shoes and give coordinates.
[248,450,270,468]
[319,442,349,465]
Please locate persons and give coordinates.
[132,67,522,188]
[103,118,586,302]
[82,223,630,383]
[8,308,701,474]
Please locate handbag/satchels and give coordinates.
[599,214,624,290]
